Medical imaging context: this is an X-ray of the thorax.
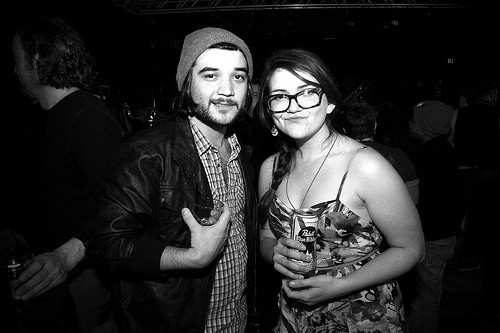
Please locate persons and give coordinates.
[225,79,262,172]
[442,76,500,333]
[256,48,426,333]
[340,99,420,206]
[393,100,478,333]
[0,14,129,333]
[85,26,261,333]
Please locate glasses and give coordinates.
[266,88,324,113]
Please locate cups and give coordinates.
[186,197,225,248]
[2,250,38,286]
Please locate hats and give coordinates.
[175,27,253,91]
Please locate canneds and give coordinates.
[289,209,318,272]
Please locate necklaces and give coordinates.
[286,133,338,210]
